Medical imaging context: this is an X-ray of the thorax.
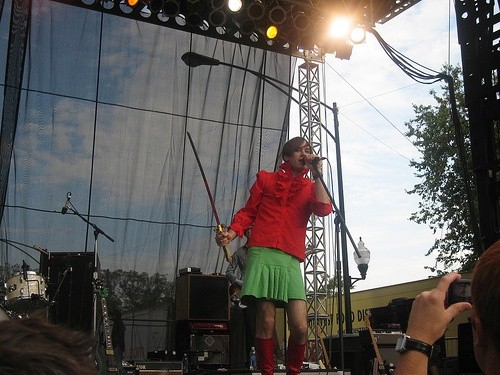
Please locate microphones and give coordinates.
[215,350,224,354]
[62,194,72,214]
[301,157,327,162]
[32,245,48,255]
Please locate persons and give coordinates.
[215,137,332,375]
[394,239,500,375]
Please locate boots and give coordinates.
[255,334,275,375]
[286,336,307,375]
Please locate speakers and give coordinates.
[174,332,232,368]
[376,345,401,367]
[38,251,96,332]
[175,274,230,322]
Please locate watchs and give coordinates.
[395,334,433,359]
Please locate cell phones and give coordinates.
[446,279,473,306]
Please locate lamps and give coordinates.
[82,0,366,60]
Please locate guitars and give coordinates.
[229,244,315,311]
[365,315,396,375]
[317,326,337,369]
[92,279,127,375]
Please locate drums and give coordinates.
[0,305,29,320]
[3,271,49,315]
